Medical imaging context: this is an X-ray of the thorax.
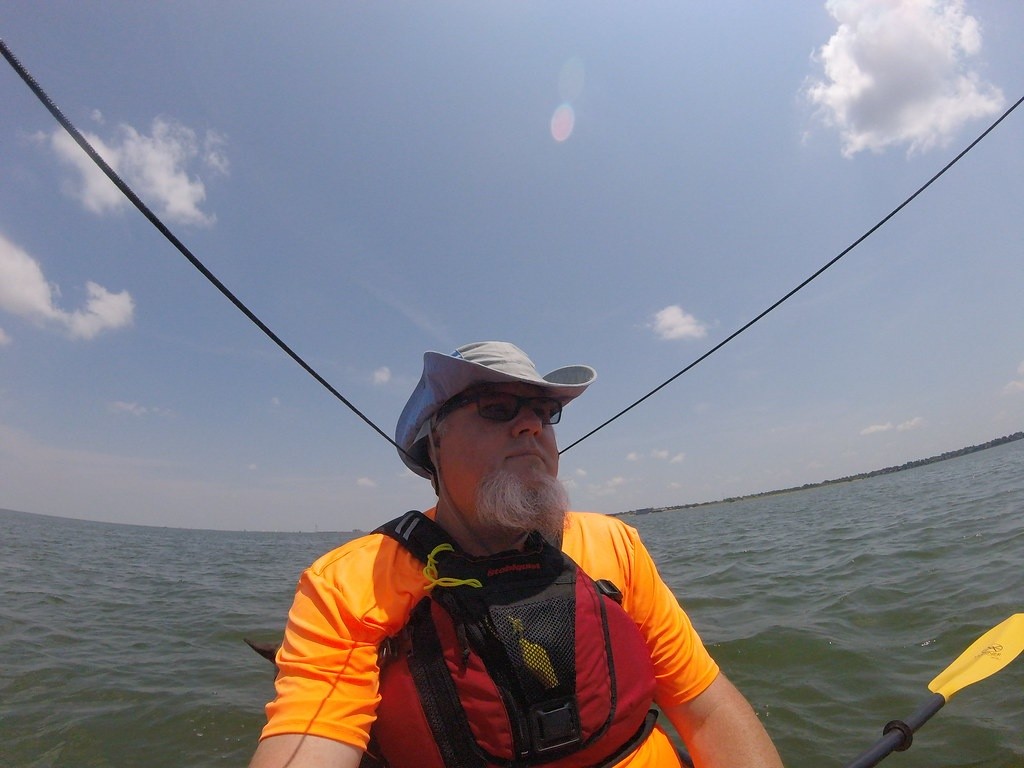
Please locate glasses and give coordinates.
[441,392,562,425]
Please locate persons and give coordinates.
[248,341,784,767]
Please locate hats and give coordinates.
[395,340,597,480]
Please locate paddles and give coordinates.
[843,611,1024,768]
